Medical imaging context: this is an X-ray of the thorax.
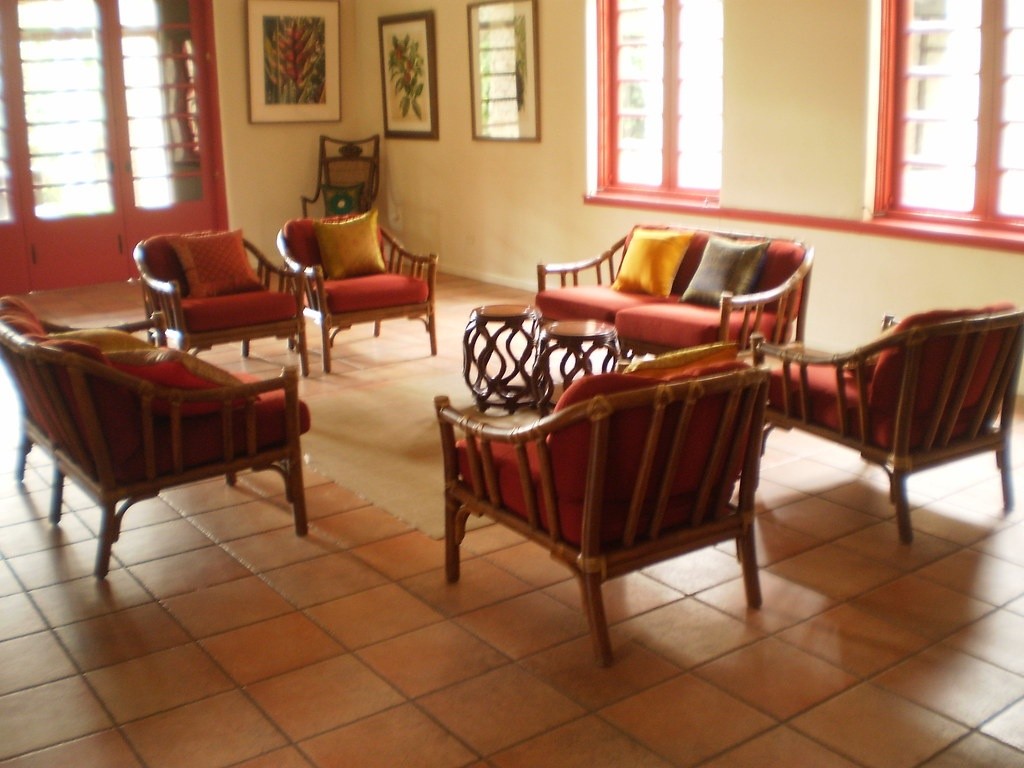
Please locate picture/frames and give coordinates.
[466,0,542,144]
[245,0,343,124]
[377,10,439,141]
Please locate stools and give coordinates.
[530,318,622,418]
[462,304,549,415]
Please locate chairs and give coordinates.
[745,301,1024,546]
[301,134,380,219]
[133,232,310,379]
[435,341,772,670]
[277,215,437,377]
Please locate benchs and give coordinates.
[0,295,313,582]
[535,224,814,359]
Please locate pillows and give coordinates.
[312,208,387,281]
[611,227,694,299]
[166,228,266,299]
[321,182,364,217]
[35,338,229,388]
[680,235,771,310]
